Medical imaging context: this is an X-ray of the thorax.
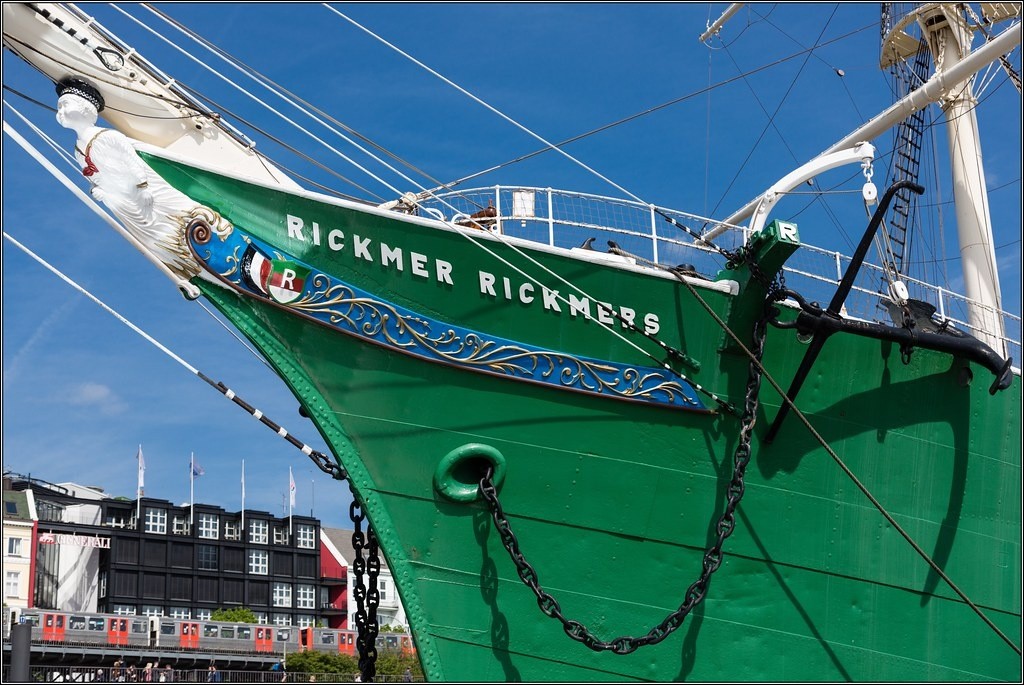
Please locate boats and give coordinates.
[2,2,1024,685]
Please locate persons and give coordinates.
[207,666,221,682]
[403,666,412,682]
[55,79,231,281]
[310,676,315,682]
[272,659,286,682]
[354,671,361,682]
[95,658,176,682]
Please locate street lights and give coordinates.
[375,612,415,665]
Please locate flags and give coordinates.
[136,449,145,496]
[189,458,204,479]
[290,472,296,508]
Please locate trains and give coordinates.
[4,606,418,659]
